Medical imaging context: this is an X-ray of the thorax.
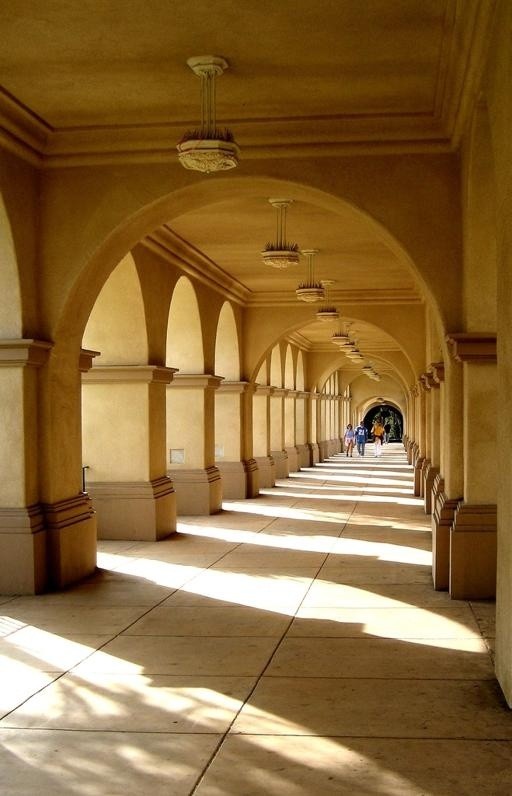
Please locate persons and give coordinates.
[344,417,391,457]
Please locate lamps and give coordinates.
[175,55,240,172]
[296,246,323,306]
[316,280,339,324]
[261,196,302,267]
[333,322,381,383]
